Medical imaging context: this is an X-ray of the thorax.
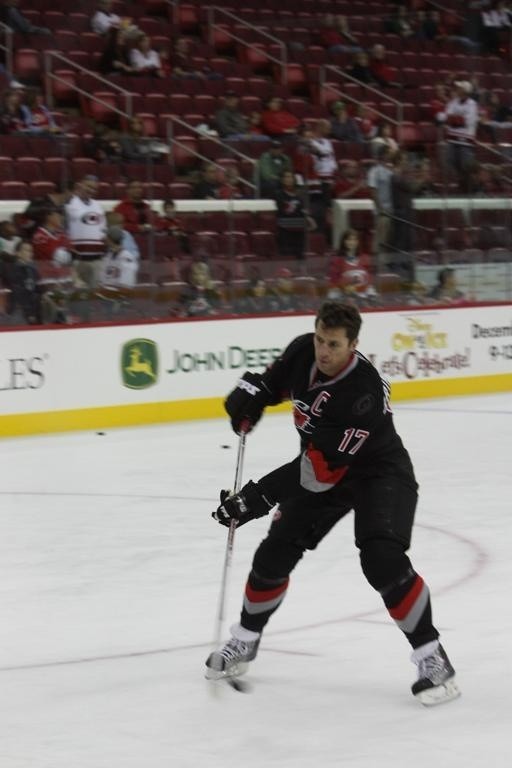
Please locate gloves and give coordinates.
[224,370,267,438]
[211,480,270,529]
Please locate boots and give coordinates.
[207,626,261,672]
[409,639,455,697]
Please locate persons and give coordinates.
[2,2,512,328]
[205,303,456,696]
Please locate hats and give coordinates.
[457,80,475,90]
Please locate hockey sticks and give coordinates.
[209,416,255,694]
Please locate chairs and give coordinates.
[0,1,512,326]
